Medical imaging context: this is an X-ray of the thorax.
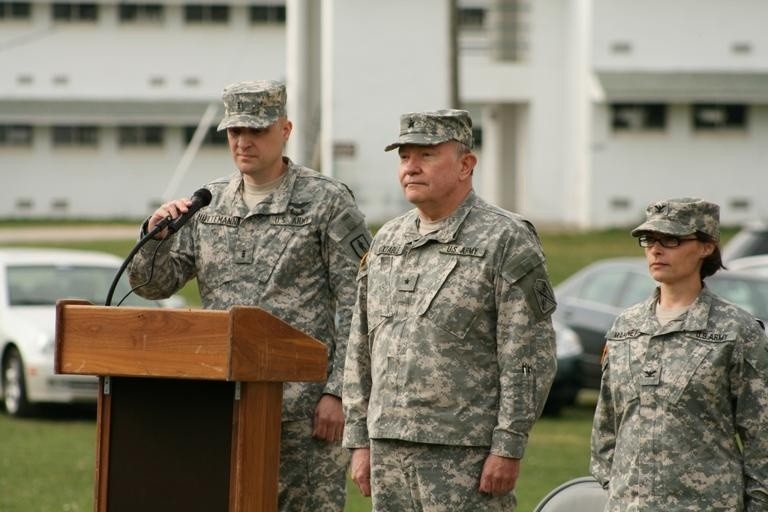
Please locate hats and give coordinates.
[216,80,287,131]
[632,197,721,240]
[384,108,473,152]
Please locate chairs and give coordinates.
[533,477,615,512]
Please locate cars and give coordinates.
[0,249,188,415]
[722,220,768,275]
[540,255,767,418]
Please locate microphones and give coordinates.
[167,188,213,235]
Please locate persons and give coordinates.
[127,78,371,512]
[341,109,559,512]
[589,196,767,512]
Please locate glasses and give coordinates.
[639,234,698,248]
